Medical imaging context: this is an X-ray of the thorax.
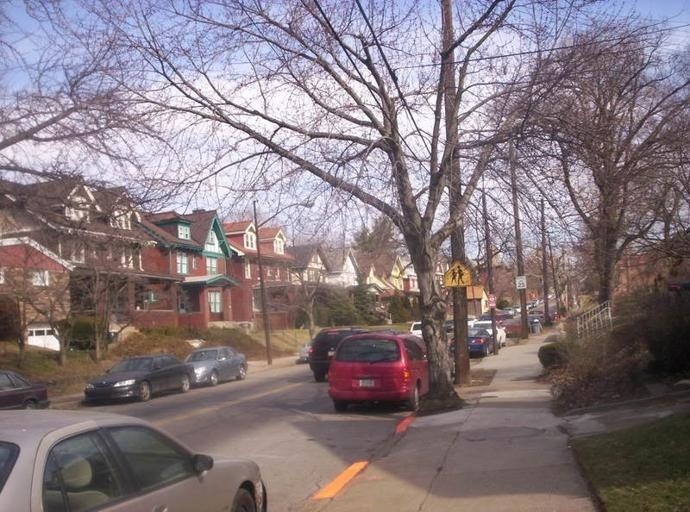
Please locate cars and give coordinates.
[0,369,51,411]
[301,294,567,413]
[84,355,197,404]
[184,346,248,386]
[0,409,269,512]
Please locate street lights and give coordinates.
[253,200,316,365]
